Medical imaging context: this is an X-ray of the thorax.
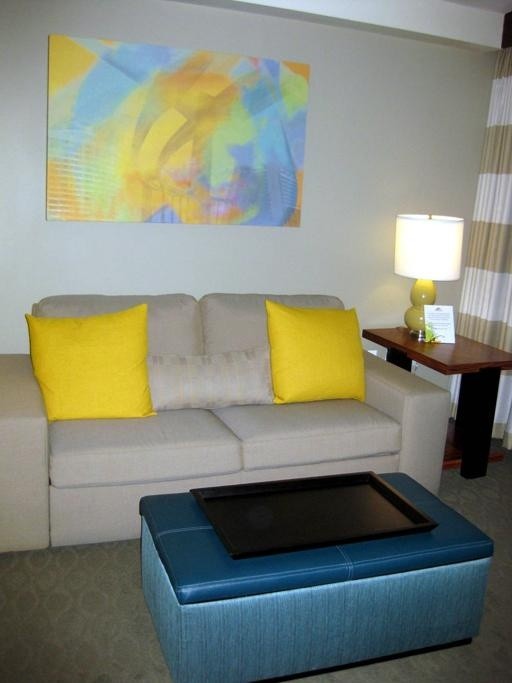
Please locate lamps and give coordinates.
[393,212,465,333]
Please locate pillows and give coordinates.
[147,352,270,412]
[264,301,367,407]
[24,303,159,424]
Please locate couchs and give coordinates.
[1,293,451,554]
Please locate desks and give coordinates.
[361,326,511,481]
